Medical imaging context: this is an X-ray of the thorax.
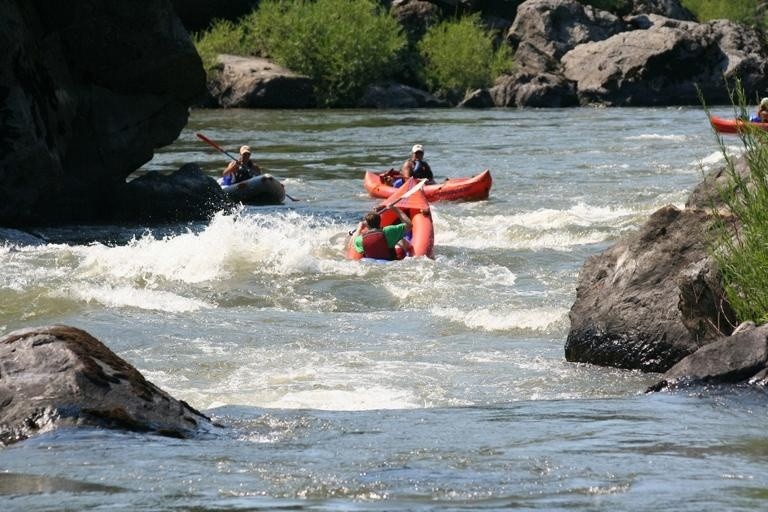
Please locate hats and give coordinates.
[412,144,424,154]
[240,145,252,155]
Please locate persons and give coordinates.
[221,144,259,185]
[354,202,414,261]
[403,142,439,187]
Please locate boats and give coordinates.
[364,169,492,201]
[217,173,285,206]
[710,115,768,134]
[346,176,434,265]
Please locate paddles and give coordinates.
[197,134,299,201]
[349,178,427,236]
